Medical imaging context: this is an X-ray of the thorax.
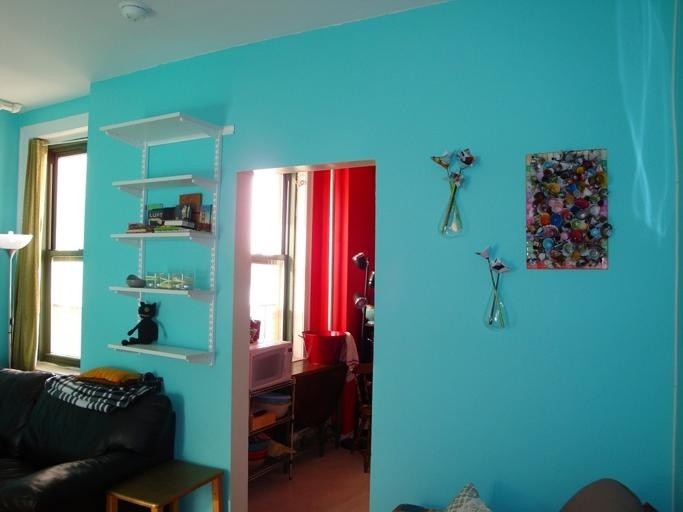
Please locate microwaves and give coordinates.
[250,338,293,391]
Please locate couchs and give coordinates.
[0,368,177,512]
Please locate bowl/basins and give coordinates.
[254,394,291,419]
[248,439,267,470]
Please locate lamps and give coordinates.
[0,229,36,371]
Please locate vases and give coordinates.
[483,286,508,333]
[437,200,463,240]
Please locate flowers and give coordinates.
[475,245,511,328]
[430,147,474,234]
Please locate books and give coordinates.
[125,194,211,234]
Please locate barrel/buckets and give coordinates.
[302,329,346,367]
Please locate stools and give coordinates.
[104,458,223,512]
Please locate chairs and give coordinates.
[351,363,373,473]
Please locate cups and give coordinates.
[146,272,194,288]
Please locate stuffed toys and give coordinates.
[121,302,157,345]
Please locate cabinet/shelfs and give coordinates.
[98,111,236,368]
[248,375,296,489]
